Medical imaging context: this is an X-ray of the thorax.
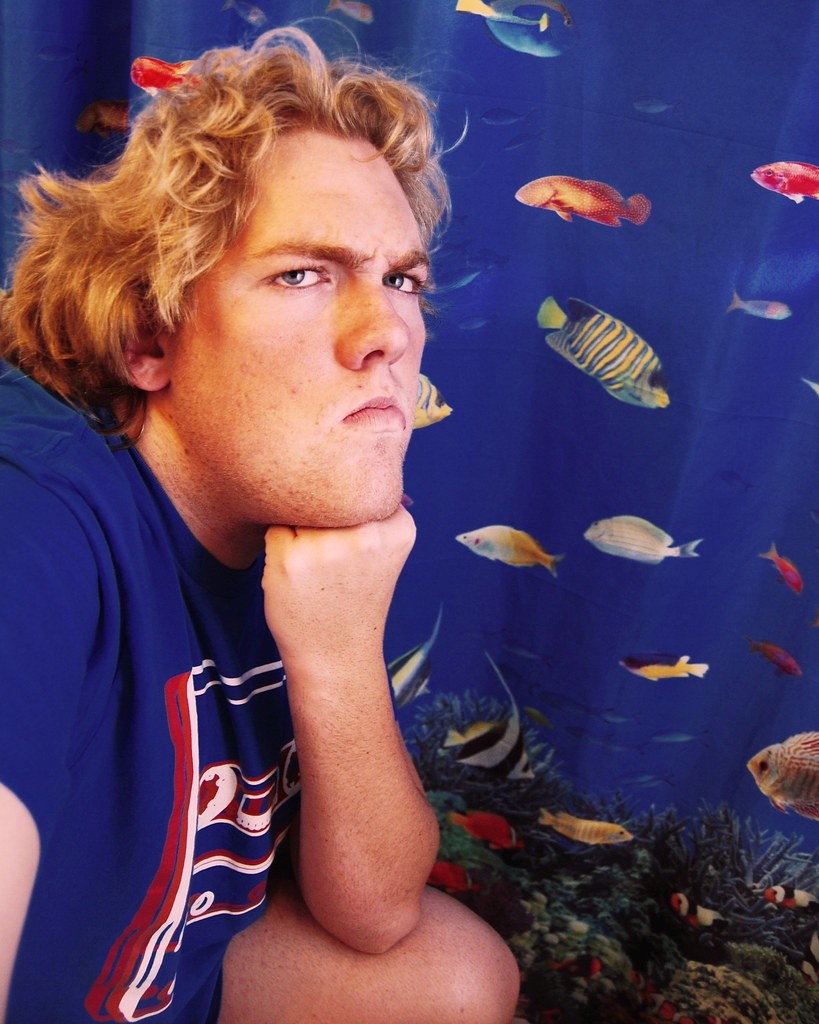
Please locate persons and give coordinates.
[0,39,519,1024]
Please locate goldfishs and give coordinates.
[77,0,818,930]
